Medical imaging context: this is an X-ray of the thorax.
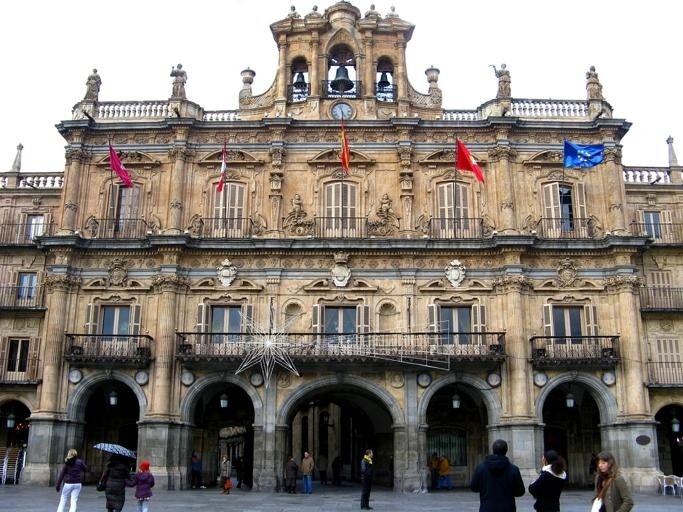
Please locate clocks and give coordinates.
[327,99,357,120]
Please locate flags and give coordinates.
[563,140,604,169]
[110,144,132,189]
[340,127,351,176]
[455,140,486,185]
[217,151,227,192]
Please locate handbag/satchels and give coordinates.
[224,480,232,490]
[591,498,602,512]
[96,482,106,491]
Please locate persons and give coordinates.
[191,450,207,489]
[379,193,394,218]
[98,453,130,511]
[288,193,302,219]
[125,462,154,512]
[170,63,187,98]
[235,456,244,488]
[589,455,597,488]
[221,454,232,494]
[528,451,568,512]
[470,439,525,512]
[429,452,454,491]
[14,422,26,448]
[56,448,88,512]
[84,69,101,101]
[592,451,633,512]
[493,63,511,97]
[585,64,599,99]
[360,449,374,509]
[284,450,344,494]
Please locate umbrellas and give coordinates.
[94,443,137,458]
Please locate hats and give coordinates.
[138,461,150,471]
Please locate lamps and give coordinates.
[7,414,16,428]
[672,418,680,432]
[219,394,229,408]
[565,392,575,407]
[451,394,461,409]
[109,391,118,405]
